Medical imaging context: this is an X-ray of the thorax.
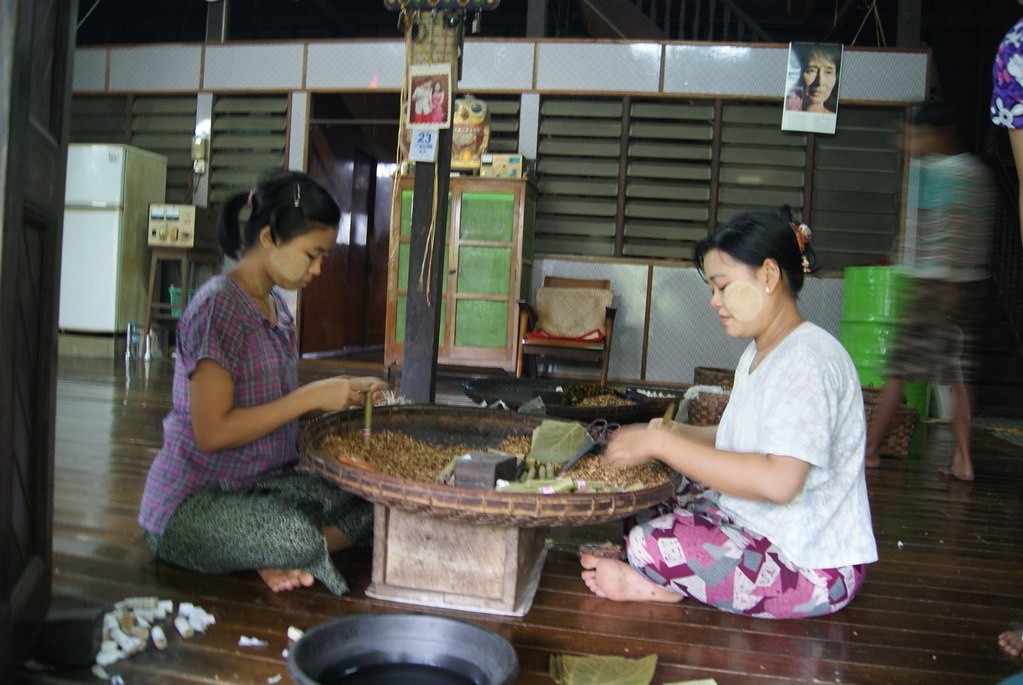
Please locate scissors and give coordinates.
[559,418,622,473]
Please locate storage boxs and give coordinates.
[365,506,550,619]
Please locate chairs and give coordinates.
[516,275,616,386]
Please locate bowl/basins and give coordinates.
[286,612,519,685]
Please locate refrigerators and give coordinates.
[59,143,167,334]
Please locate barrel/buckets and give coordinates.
[839,265,932,458]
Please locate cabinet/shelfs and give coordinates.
[384,173,538,383]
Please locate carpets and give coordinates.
[972,417,1023,447]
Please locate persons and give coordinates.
[139,172,389,591]
[791,44,842,114]
[991,21,1023,657]
[411,78,445,122]
[865,110,995,480]
[580,212,879,619]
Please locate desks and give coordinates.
[142,246,220,359]
